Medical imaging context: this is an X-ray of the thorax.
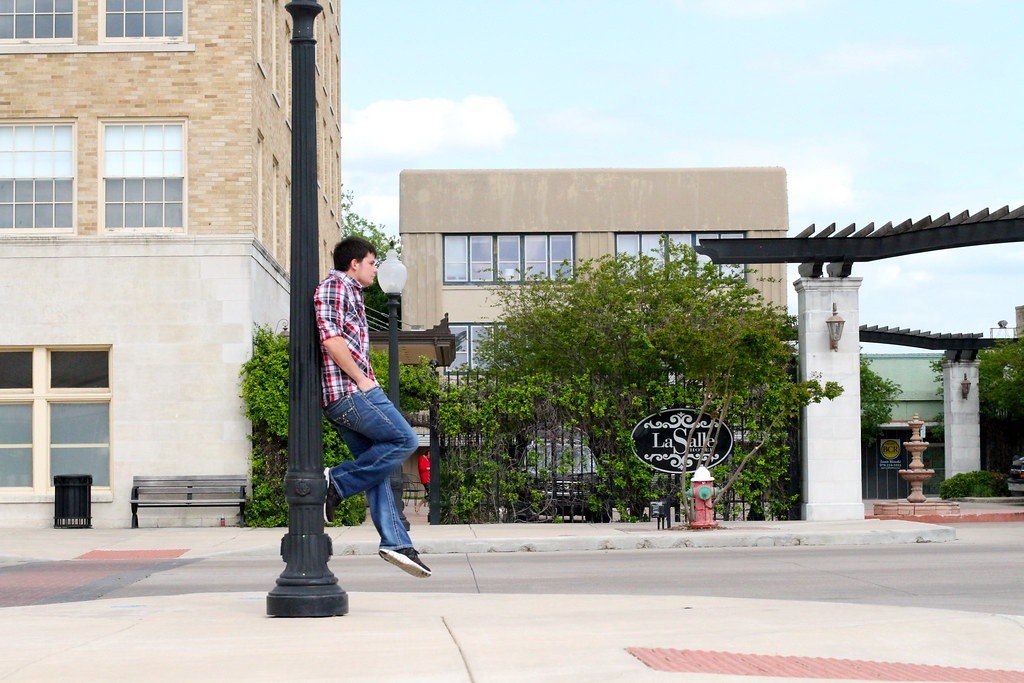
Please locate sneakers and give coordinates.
[378,547,432,578]
[324,467,343,523]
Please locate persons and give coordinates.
[313,237,431,579]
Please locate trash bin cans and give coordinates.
[53,474,93,529]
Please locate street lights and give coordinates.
[375,242,415,535]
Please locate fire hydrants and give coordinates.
[685,466,718,531]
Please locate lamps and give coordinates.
[962,373,971,399]
[826,303,846,351]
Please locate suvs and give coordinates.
[521,439,611,523]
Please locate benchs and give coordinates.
[129,475,249,527]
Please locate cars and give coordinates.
[1010,457,1024,480]
